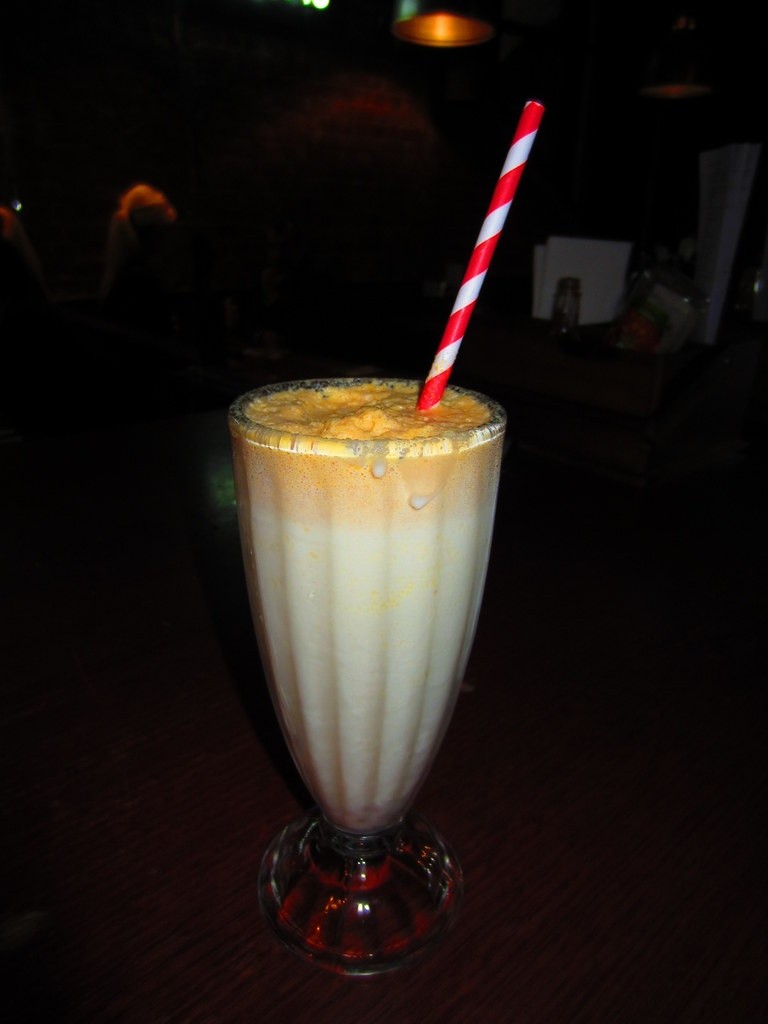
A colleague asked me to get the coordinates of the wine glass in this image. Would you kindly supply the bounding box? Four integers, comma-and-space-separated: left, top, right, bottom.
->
227, 376, 506, 978
